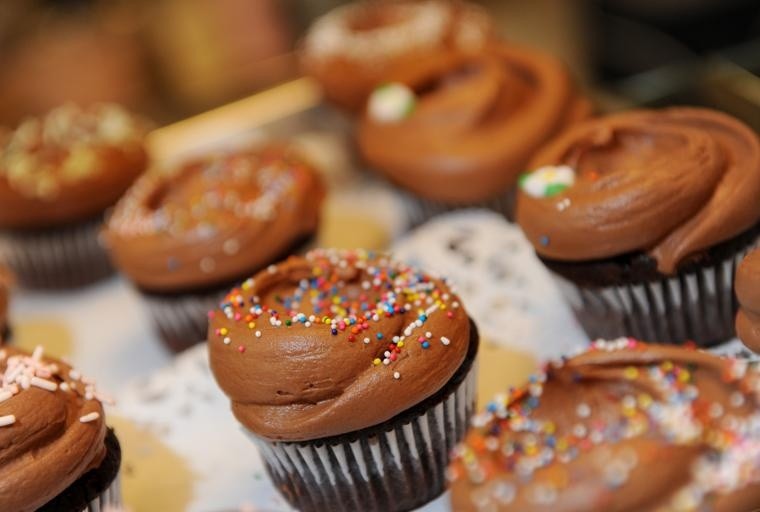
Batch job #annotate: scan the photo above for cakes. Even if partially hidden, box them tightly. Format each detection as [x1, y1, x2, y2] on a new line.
[1, 0, 760, 511]
